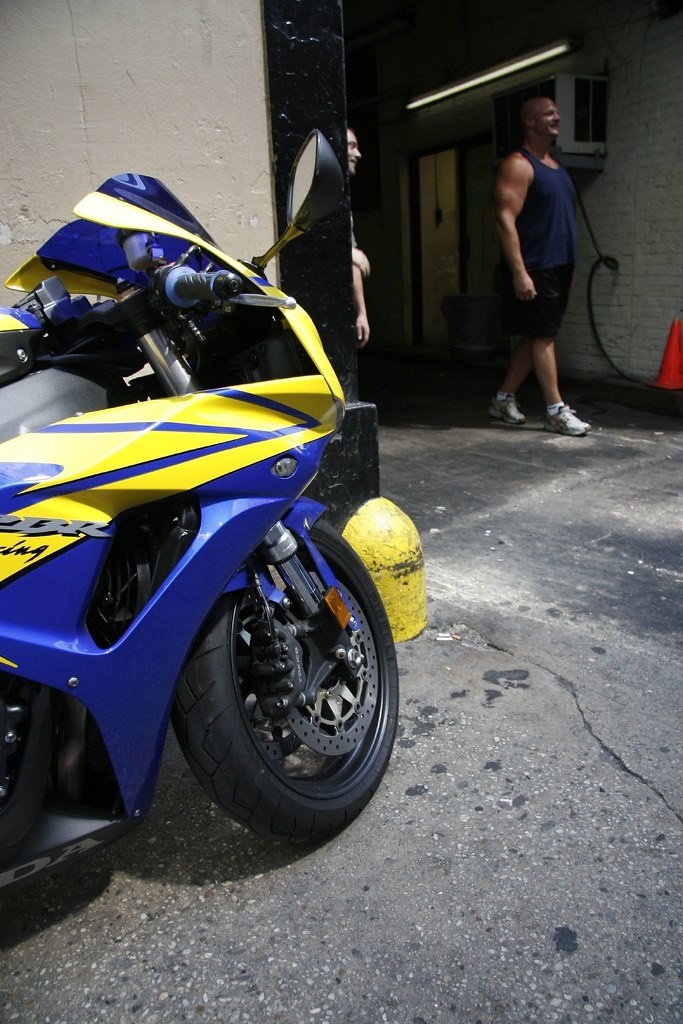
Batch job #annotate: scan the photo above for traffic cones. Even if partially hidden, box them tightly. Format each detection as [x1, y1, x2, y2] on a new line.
[646, 318, 683, 391]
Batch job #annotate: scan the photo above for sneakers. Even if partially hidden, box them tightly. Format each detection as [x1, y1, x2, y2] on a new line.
[488, 395, 526, 424]
[545, 404, 592, 435]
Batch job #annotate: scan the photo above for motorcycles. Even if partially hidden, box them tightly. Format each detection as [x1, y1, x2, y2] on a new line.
[0, 130, 400, 898]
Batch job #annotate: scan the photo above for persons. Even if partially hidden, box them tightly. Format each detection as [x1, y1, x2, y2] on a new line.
[490, 97, 593, 435]
[346, 125, 372, 348]
